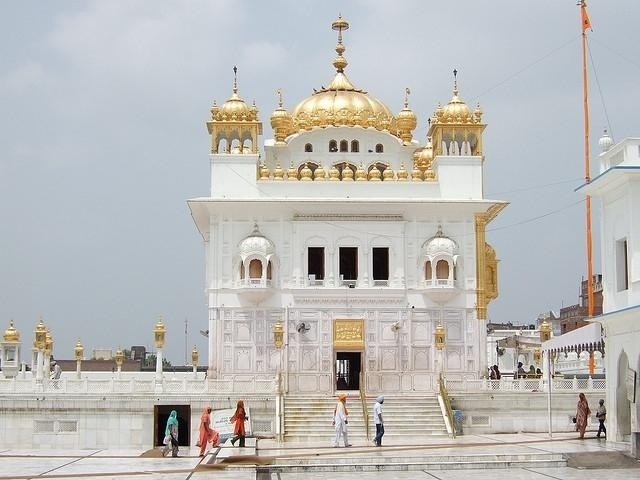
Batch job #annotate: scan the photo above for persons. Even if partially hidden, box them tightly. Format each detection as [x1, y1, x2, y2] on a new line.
[596, 398, 606, 438]
[333, 393, 352, 447]
[372, 394, 385, 448]
[50, 361, 62, 389]
[488, 362, 541, 389]
[230, 400, 248, 448]
[576, 393, 589, 439]
[195, 406, 220, 458]
[160, 411, 180, 458]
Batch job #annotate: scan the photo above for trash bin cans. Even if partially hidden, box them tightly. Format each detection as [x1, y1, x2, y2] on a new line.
[451, 409, 464, 436]
